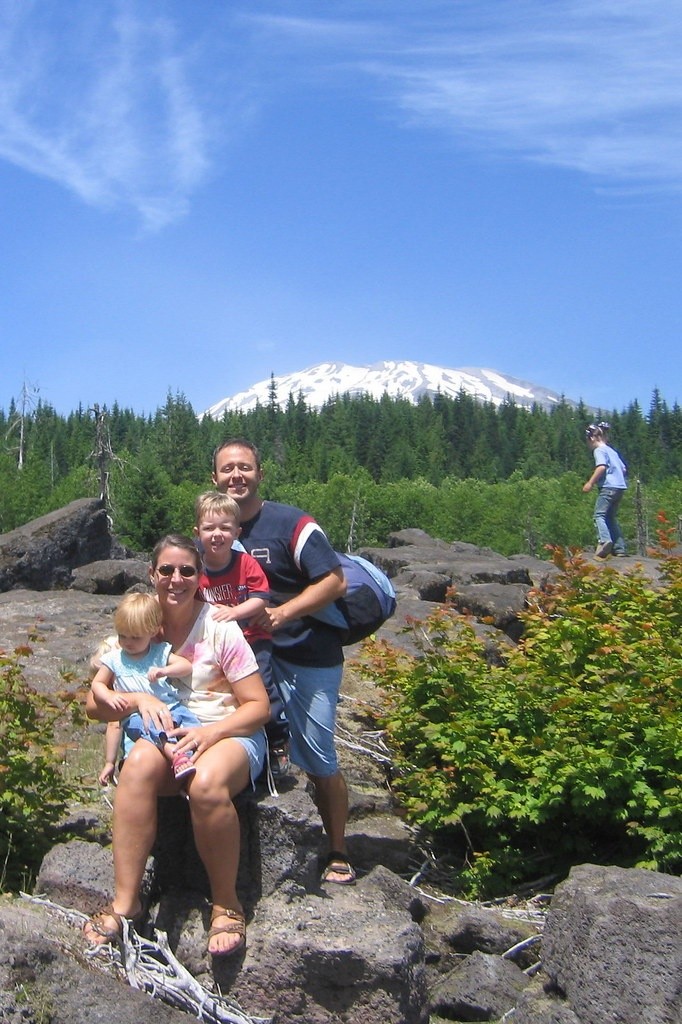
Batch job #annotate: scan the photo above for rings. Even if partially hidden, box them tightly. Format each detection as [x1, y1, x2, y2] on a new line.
[194, 741, 197, 747]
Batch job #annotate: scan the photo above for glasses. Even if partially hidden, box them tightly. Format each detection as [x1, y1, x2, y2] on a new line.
[153, 564, 198, 578]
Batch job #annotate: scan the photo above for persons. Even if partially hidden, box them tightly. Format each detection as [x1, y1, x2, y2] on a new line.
[87, 533, 272, 956]
[194, 492, 269, 694]
[194, 441, 350, 885]
[581, 422, 628, 557]
[92, 592, 203, 777]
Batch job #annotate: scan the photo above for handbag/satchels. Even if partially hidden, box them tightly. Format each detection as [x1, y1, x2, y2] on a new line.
[334, 551, 396, 646]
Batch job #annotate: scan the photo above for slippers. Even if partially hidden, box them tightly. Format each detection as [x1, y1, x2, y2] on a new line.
[83, 897, 147, 944]
[207, 908, 246, 957]
[321, 851, 356, 885]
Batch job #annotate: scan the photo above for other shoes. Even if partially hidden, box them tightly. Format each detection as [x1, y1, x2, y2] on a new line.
[613, 543, 626, 556]
[595, 542, 613, 558]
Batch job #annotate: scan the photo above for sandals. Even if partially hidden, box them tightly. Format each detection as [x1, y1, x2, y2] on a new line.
[270, 745, 288, 778]
[172, 754, 196, 780]
